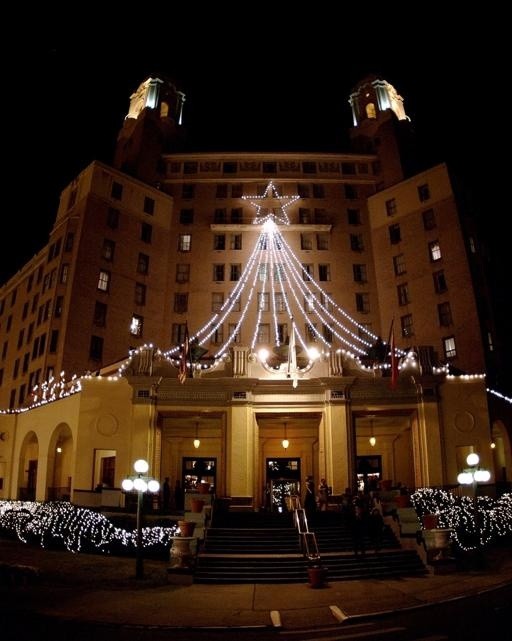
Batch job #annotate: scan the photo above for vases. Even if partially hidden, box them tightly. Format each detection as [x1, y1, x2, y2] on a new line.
[394, 495, 410, 508]
[306, 568, 331, 590]
[421, 513, 440, 530]
[197, 482, 211, 496]
[176, 520, 198, 537]
[190, 498, 205, 515]
[379, 478, 394, 492]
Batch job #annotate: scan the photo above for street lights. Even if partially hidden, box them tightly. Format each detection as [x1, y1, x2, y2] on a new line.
[457, 452, 490, 573]
[122, 459, 162, 578]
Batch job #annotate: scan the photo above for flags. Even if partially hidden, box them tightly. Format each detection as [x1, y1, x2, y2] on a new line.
[387, 326, 400, 389]
[178, 326, 190, 384]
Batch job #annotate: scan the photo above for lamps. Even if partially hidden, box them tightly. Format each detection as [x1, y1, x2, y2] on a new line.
[193, 422, 201, 449]
[366, 419, 379, 449]
[56, 440, 65, 454]
[489, 433, 497, 451]
[280, 422, 292, 453]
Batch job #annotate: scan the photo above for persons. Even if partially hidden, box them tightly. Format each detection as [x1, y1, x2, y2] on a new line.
[303, 475, 316, 511]
[318, 478, 334, 511]
